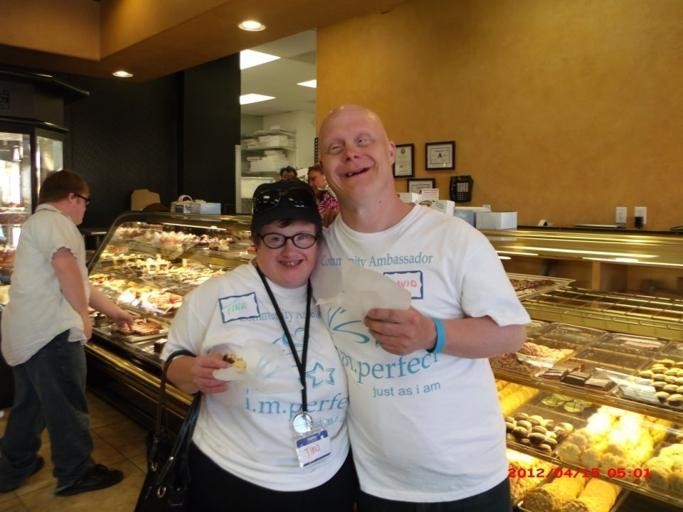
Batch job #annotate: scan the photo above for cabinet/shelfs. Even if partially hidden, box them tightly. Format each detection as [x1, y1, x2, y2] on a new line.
[241, 130, 297, 177]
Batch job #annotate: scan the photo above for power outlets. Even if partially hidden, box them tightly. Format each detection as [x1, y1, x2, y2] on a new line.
[635, 207, 647, 224]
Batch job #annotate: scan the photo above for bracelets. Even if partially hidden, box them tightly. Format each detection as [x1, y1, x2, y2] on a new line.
[427, 317, 445, 354]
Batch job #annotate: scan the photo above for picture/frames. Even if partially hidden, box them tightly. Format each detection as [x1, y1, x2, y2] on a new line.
[425, 141, 455, 170]
[393, 143, 414, 177]
[407, 178, 435, 193]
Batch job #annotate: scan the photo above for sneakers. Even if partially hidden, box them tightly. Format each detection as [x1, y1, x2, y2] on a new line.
[56, 464, 123, 496]
[0, 456, 45, 492]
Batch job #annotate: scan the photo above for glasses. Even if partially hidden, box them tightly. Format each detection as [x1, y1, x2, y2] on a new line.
[255, 189, 312, 211]
[258, 231, 320, 249]
[74, 193, 92, 206]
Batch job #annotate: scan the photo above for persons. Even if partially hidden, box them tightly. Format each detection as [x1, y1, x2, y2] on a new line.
[1, 172, 134, 498]
[308, 165, 340, 225]
[308, 105, 531, 512]
[280, 167, 297, 181]
[161, 177, 360, 512]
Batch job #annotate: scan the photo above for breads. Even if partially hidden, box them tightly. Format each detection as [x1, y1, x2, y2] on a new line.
[505, 359, 683, 512]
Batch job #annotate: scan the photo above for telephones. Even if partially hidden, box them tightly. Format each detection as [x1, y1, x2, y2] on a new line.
[449, 175, 473, 202]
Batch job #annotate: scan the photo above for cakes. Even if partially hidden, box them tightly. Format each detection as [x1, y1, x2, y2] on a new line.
[222, 354, 247, 372]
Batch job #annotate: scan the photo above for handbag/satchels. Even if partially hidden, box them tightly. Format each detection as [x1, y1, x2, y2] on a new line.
[134, 430, 189, 512]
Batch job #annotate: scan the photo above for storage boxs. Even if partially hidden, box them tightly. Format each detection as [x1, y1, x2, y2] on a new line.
[476, 212, 518, 229]
[454, 207, 489, 227]
[192, 203, 221, 214]
[396, 193, 421, 202]
[170, 200, 206, 213]
[416, 200, 455, 216]
